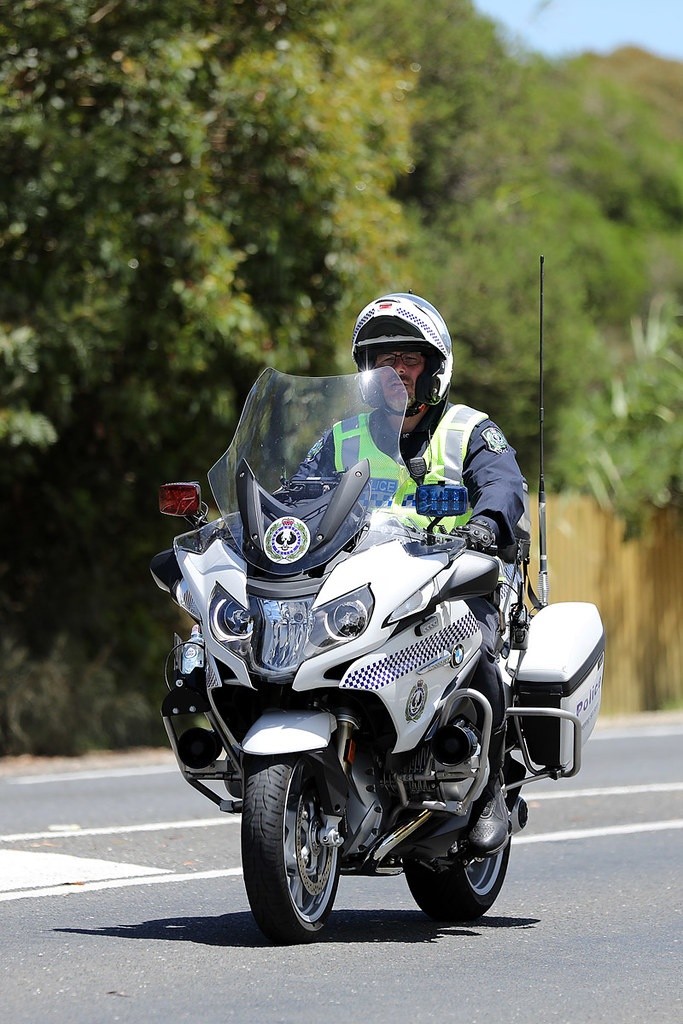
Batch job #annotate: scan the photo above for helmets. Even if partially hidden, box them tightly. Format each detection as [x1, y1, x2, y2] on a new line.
[350, 290, 453, 417]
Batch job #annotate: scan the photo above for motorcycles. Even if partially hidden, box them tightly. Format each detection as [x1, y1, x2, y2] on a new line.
[146, 252, 608, 947]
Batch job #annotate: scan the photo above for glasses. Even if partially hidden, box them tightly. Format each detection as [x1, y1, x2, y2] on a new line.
[372, 352, 423, 368]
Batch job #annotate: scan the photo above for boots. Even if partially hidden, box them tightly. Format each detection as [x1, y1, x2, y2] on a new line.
[467, 712, 514, 857]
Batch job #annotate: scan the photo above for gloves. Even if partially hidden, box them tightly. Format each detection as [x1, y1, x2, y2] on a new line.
[444, 516, 498, 553]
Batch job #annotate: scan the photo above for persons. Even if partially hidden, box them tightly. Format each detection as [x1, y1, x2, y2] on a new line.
[282, 289, 528, 858]
